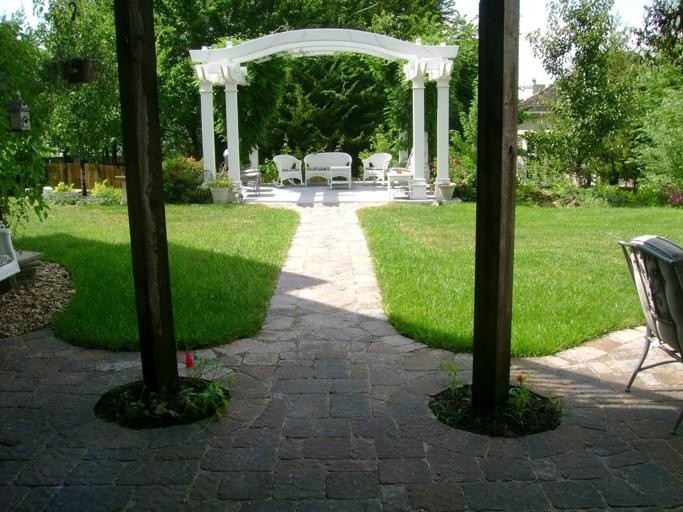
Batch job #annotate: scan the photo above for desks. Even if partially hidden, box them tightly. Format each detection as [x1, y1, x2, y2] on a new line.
[114, 176, 127, 206]
[328, 165, 352, 190]
[386, 170, 413, 201]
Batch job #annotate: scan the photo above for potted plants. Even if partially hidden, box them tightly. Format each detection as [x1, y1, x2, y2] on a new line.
[208, 177, 232, 204]
[439, 180, 458, 200]
[39, 22, 112, 84]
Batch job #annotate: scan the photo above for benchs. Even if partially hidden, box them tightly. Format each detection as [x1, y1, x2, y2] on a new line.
[303, 151, 353, 187]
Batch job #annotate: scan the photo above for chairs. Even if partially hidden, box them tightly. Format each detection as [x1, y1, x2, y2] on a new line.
[270, 154, 305, 188]
[360, 152, 393, 188]
[0, 226, 21, 282]
[219, 149, 261, 197]
[617, 233, 683, 435]
